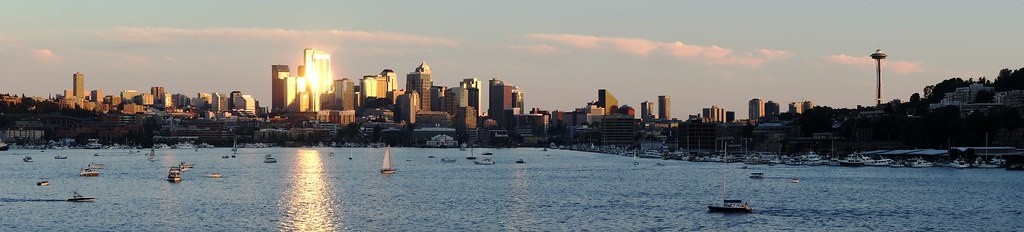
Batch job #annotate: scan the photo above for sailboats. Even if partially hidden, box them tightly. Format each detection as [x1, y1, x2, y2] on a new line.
[707, 139, 755, 215]
[379, 144, 398, 175]
[348, 148, 353, 160]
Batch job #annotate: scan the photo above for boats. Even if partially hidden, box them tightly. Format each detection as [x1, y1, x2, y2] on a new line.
[549, 141, 1024, 171]
[750, 172, 765, 179]
[791, 179, 800, 183]
[428, 146, 526, 166]
[0, 141, 277, 203]
[327, 152, 335, 157]
